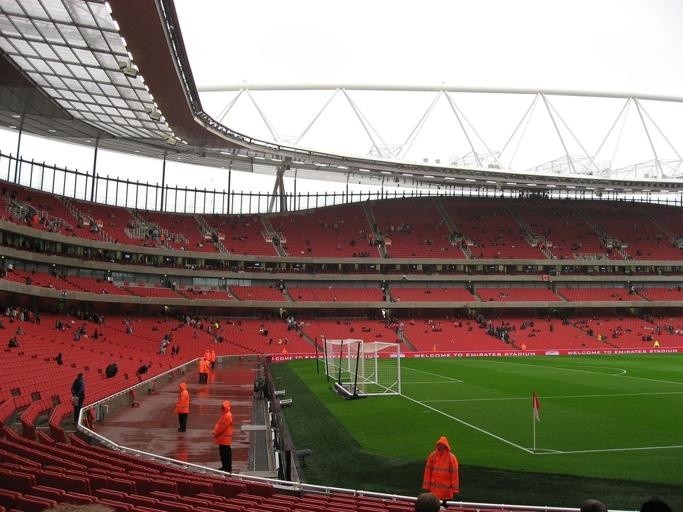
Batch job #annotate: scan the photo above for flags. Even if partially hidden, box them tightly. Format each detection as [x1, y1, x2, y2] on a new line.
[532, 389, 540, 423]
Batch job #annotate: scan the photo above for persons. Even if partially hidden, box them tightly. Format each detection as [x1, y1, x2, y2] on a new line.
[197, 358, 207, 384]
[422, 436, 460, 501]
[414, 492, 449, 512]
[212, 399, 232, 477]
[640, 497, 674, 511]
[578, 499, 609, 511]
[203, 358, 209, 384]
[173, 382, 190, 432]
[69, 372, 87, 426]
[1, 186, 682, 379]
